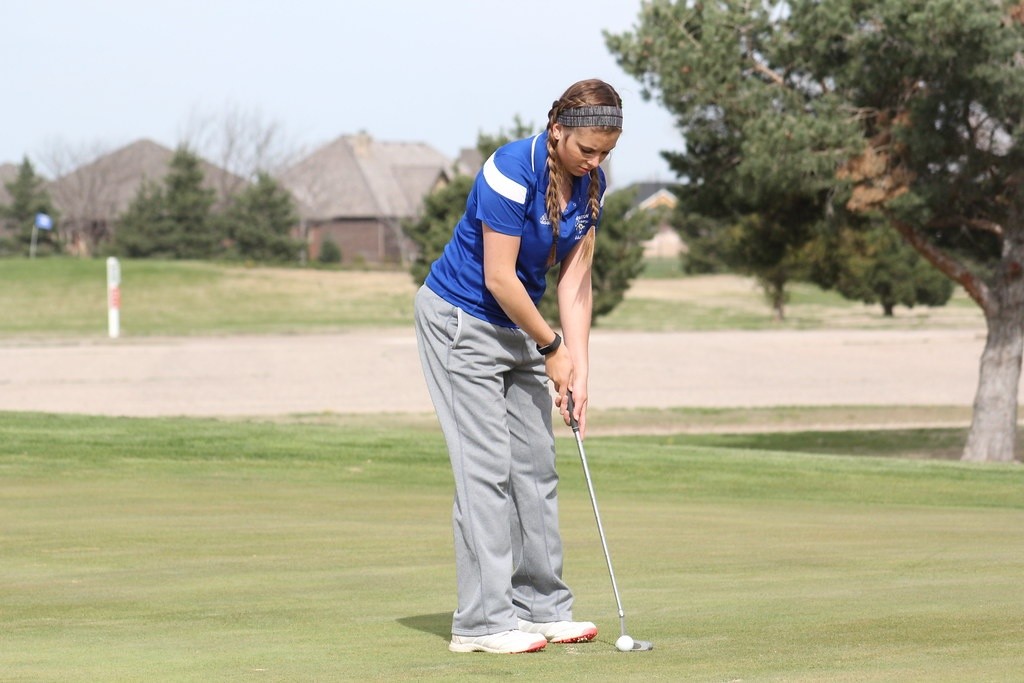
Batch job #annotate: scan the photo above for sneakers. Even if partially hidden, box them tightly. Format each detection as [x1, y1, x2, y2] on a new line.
[448, 629, 548, 654]
[517, 618, 598, 644]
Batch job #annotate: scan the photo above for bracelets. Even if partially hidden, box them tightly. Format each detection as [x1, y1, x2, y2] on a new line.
[536, 332, 561, 355]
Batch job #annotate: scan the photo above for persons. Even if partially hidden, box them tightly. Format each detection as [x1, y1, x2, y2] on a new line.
[413, 79, 623, 653]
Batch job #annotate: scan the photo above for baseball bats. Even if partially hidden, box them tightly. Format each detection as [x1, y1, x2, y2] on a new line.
[560, 377, 655, 654]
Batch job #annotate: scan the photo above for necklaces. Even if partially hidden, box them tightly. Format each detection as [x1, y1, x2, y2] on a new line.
[559, 185, 566, 203]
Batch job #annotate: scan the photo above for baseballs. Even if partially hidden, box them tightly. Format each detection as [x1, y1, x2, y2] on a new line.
[615, 634, 634, 653]
